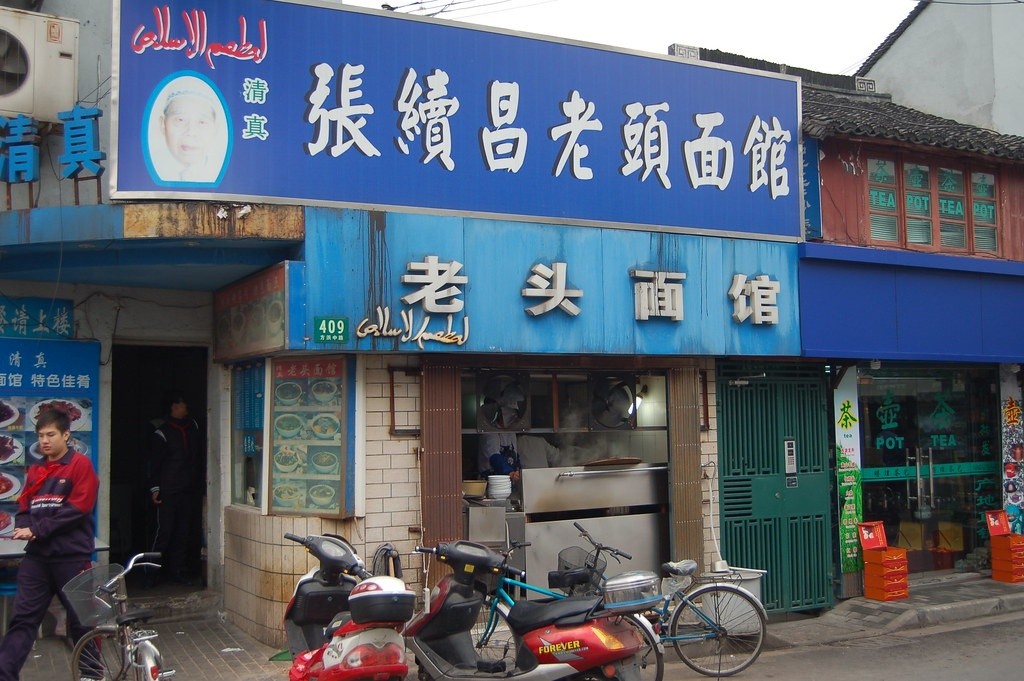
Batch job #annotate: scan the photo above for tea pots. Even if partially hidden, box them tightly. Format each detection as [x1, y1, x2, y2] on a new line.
[1004, 445, 1023, 505]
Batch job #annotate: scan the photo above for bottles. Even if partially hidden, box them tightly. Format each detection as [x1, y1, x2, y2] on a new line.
[866, 493, 873, 513]
[882, 494, 889, 512]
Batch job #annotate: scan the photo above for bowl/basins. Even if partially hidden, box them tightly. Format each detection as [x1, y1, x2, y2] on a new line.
[463, 480, 487, 496]
[274, 450, 299, 472]
[312, 381, 337, 401]
[275, 414, 303, 438]
[488, 475, 512, 499]
[275, 382, 302, 405]
[312, 413, 340, 438]
[311, 452, 337, 473]
[309, 485, 335, 505]
[273, 485, 301, 506]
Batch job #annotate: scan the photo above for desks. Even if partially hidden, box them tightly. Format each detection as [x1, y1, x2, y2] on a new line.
[0, 537, 110, 557]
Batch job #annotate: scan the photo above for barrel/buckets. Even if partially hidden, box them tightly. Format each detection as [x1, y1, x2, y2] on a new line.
[700, 571, 763, 635]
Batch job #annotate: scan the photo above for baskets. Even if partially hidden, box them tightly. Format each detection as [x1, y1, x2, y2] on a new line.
[62, 563, 127, 627]
[557, 546, 607, 598]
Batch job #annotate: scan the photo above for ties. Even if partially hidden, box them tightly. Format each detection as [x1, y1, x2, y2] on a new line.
[166, 419, 192, 450]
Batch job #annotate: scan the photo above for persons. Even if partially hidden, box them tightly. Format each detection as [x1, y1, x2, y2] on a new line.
[477, 376, 560, 486]
[142, 393, 199, 590]
[0, 409, 104, 681]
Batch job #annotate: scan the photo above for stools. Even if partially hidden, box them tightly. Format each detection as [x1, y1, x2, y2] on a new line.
[0, 568, 42, 640]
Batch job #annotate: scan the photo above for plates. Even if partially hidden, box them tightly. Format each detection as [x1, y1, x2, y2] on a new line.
[29, 399, 87, 431]
[0, 435, 23, 464]
[0, 473, 21, 499]
[0, 402, 20, 429]
[30, 437, 88, 459]
[0, 517, 15, 534]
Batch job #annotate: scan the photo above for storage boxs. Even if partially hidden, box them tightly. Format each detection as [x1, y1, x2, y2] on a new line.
[857, 521, 908, 601]
[985, 510, 1024, 583]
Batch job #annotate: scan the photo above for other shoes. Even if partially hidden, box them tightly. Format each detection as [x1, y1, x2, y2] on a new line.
[164, 567, 185, 585]
[131, 574, 164, 590]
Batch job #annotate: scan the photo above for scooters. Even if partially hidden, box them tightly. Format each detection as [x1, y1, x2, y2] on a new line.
[281, 531, 418, 680]
[402, 539, 665, 681]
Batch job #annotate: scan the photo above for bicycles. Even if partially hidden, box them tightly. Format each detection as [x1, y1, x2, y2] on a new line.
[470, 538, 666, 681]
[556, 521, 768, 681]
[60, 551, 177, 681]
